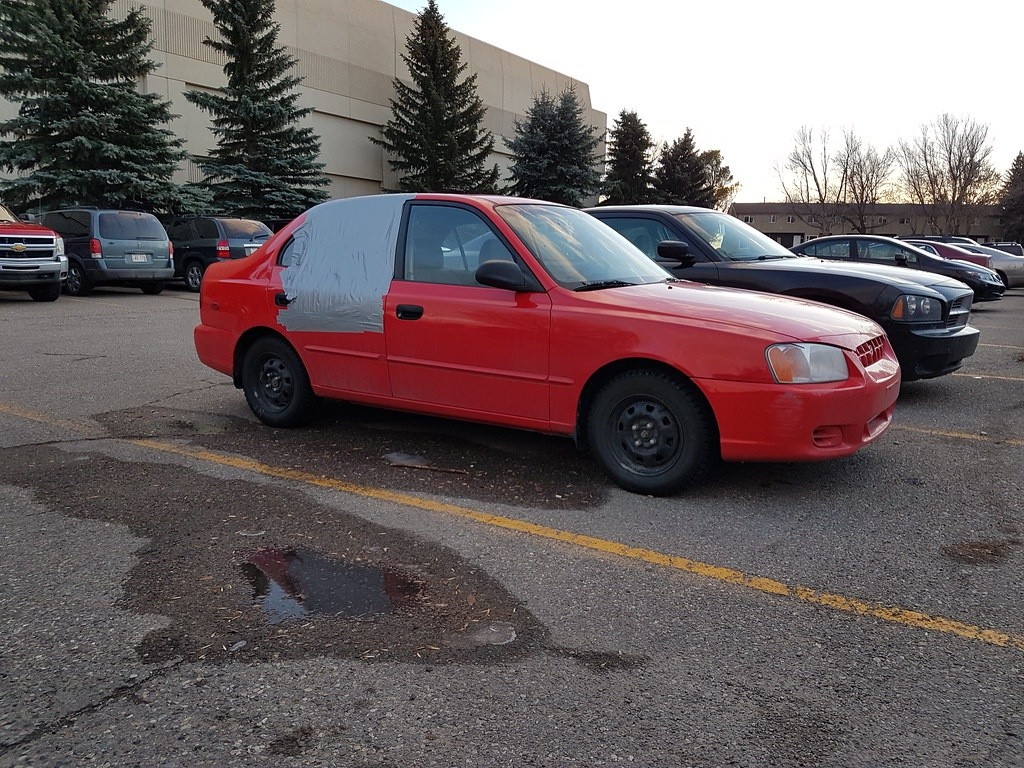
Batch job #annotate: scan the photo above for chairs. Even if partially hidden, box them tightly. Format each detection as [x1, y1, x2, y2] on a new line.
[633, 235, 650, 254]
[414, 238, 460, 285]
[469, 238, 513, 285]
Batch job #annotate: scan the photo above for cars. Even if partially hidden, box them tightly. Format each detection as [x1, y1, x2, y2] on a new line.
[195, 193, 900, 495]
[905, 243, 1024, 289]
[843, 240, 993, 270]
[787, 235, 1006, 304]
[440, 204, 980, 382]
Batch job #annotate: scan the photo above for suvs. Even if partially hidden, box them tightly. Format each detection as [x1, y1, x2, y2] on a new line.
[167, 214, 275, 292]
[893, 236, 979, 246]
[980, 242, 1024, 256]
[25, 206, 176, 296]
[0, 201, 69, 301]
